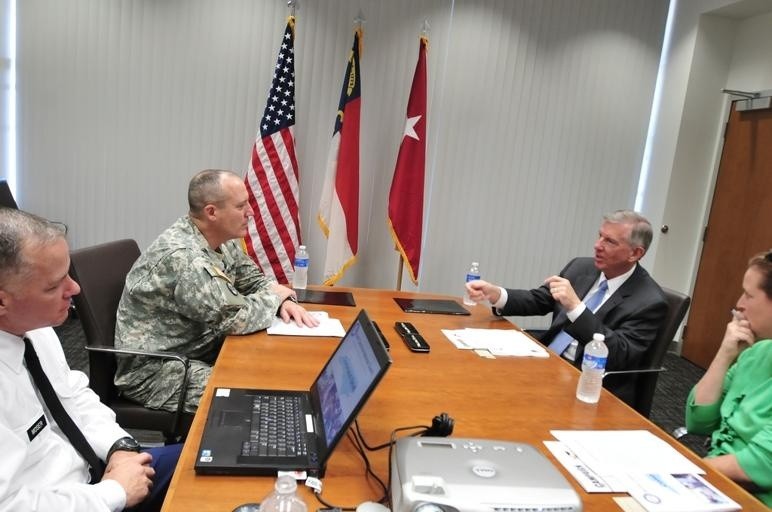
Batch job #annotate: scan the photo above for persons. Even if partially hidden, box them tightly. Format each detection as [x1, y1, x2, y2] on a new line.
[113, 169, 320, 414]
[0, 207, 184, 512]
[465, 211, 671, 406]
[684, 250, 772, 508]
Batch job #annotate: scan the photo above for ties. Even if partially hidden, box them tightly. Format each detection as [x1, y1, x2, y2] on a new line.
[24, 338, 103, 481]
[547, 280, 608, 355]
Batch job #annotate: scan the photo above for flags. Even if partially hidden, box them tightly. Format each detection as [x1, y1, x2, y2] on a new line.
[381, 21, 427, 293]
[236, 0, 302, 287]
[316, 16, 363, 288]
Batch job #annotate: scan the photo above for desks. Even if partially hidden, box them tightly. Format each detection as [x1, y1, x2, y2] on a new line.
[160, 283, 772, 512]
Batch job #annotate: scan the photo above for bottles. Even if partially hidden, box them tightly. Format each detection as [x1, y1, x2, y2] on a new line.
[259, 475, 308, 511]
[291, 245, 309, 290]
[576, 333, 608, 403]
[462, 262, 480, 306]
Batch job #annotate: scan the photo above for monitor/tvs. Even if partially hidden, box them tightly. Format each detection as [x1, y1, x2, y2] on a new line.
[193, 309, 394, 479]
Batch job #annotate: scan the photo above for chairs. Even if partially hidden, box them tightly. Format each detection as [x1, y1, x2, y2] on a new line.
[671, 425, 717, 449]
[69, 237, 195, 447]
[522, 284, 691, 421]
[0, 179, 76, 316]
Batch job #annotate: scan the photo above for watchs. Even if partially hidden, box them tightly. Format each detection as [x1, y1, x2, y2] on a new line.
[287, 294, 296, 302]
[106, 437, 141, 461]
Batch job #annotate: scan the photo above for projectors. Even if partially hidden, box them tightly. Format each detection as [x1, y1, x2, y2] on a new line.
[391, 436, 583, 511]
